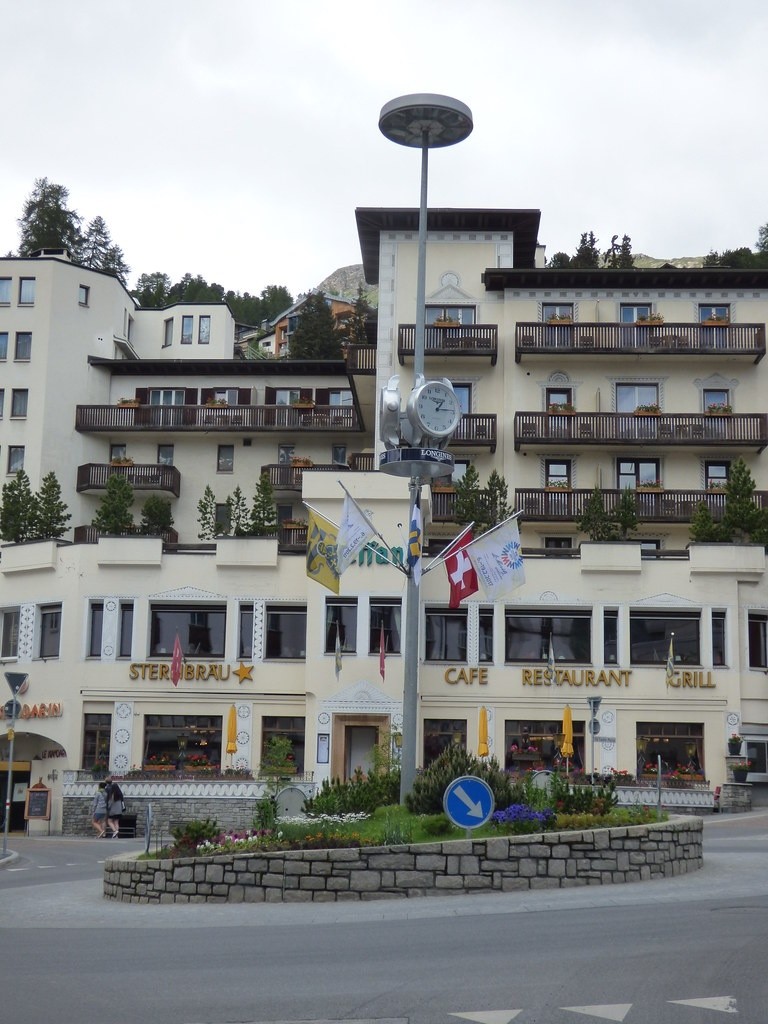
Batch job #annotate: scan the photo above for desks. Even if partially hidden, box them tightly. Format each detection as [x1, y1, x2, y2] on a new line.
[679, 500, 695, 516]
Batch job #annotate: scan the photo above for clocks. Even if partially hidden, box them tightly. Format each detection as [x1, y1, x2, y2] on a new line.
[407, 372, 462, 438]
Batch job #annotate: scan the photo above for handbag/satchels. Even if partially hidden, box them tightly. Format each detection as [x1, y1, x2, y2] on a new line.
[121, 801, 126, 812]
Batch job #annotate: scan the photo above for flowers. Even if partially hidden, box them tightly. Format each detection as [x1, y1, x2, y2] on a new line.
[434, 311, 724, 322]
[511, 734, 752, 783]
[282, 517, 307, 526]
[117, 397, 317, 406]
[144, 756, 298, 767]
[291, 456, 312, 464]
[546, 479, 727, 489]
[548, 402, 732, 411]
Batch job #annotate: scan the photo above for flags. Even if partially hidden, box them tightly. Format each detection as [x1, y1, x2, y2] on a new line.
[465, 517, 526, 602]
[333, 631, 343, 682]
[170, 638, 182, 687]
[443, 530, 479, 609]
[404, 493, 422, 587]
[305, 508, 340, 597]
[546, 643, 557, 687]
[378, 627, 386, 682]
[335, 492, 375, 576]
[665, 639, 675, 690]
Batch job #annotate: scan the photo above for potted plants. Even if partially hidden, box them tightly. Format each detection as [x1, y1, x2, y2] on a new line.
[195, 471, 280, 567]
[91, 473, 175, 566]
[111, 456, 133, 467]
[687, 454, 768, 573]
[0, 469, 73, 573]
[574, 483, 643, 571]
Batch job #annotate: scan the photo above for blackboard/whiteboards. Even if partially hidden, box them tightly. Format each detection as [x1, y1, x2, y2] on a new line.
[24, 789, 51, 820]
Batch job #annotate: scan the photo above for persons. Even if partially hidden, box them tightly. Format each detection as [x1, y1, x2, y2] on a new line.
[91, 777, 126, 839]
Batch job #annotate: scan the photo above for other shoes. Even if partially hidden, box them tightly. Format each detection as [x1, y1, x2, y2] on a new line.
[97, 830, 105, 838]
[102, 834, 106, 838]
[112, 830, 119, 838]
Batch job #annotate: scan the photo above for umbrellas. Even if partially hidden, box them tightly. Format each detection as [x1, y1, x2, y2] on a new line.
[227, 704, 238, 768]
[561, 704, 574, 773]
[477, 706, 489, 762]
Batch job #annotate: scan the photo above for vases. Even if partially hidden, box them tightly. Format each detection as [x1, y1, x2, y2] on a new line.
[144, 764, 299, 774]
[117, 403, 315, 408]
[282, 523, 308, 529]
[431, 488, 728, 494]
[547, 410, 732, 417]
[434, 319, 732, 326]
[291, 463, 313, 467]
[732, 769, 749, 783]
[728, 742, 742, 756]
